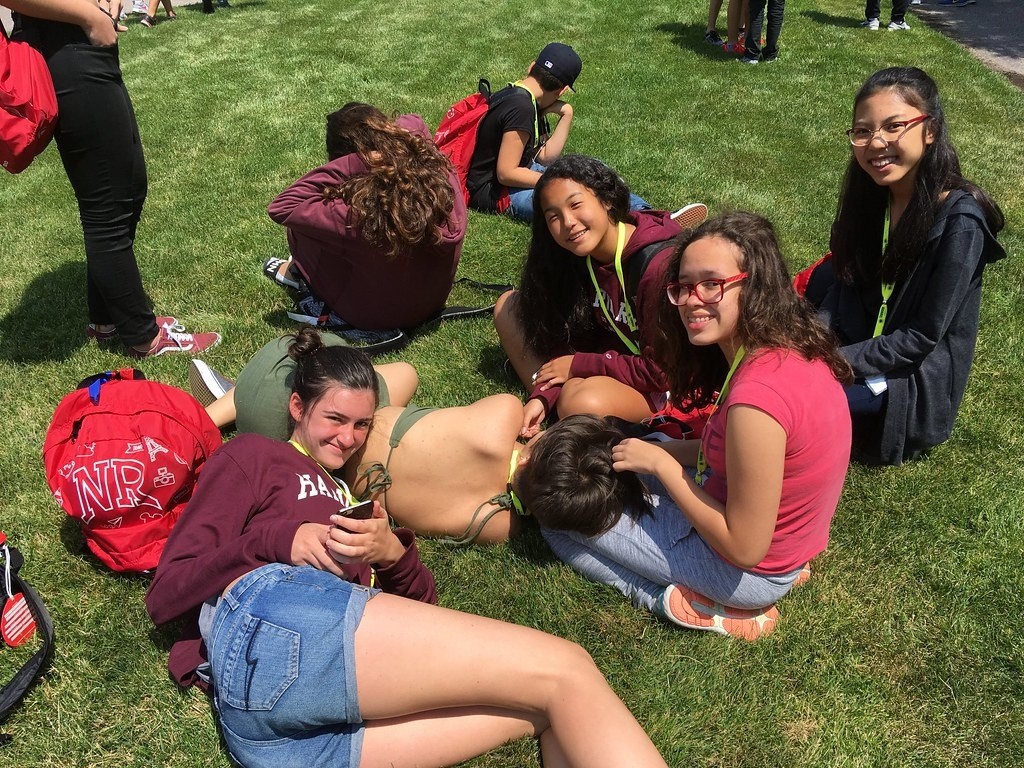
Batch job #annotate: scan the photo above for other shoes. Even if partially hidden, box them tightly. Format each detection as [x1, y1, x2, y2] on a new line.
[188, 359, 237, 407]
[860, 18, 879, 30]
[722, 41, 746, 55]
[765, 57, 777, 64]
[791, 560, 811, 584]
[740, 36, 765, 47]
[736, 56, 758, 65]
[887, 20, 910, 31]
[739, 28, 745, 34]
[661, 582, 779, 642]
[703, 30, 723, 46]
[911, 0, 921, 4]
[263, 256, 300, 291]
[670, 203, 709, 231]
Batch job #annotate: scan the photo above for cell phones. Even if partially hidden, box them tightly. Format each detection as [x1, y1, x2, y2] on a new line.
[333, 500, 375, 534]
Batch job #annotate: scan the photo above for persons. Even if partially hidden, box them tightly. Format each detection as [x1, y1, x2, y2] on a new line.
[861, 0, 913, 31]
[805, 66, 1007, 463]
[494, 156, 686, 436]
[263, 104, 469, 348]
[132, 0, 176, 28]
[542, 210, 851, 640]
[190, 332, 653, 548]
[146, 329, 668, 768]
[705, 0, 786, 65]
[465, 43, 708, 231]
[202, 0, 231, 14]
[0, 0, 221, 360]
[98, 0, 128, 31]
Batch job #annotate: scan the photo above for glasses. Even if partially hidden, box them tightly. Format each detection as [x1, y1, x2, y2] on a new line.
[665, 271, 751, 306]
[846, 114, 930, 147]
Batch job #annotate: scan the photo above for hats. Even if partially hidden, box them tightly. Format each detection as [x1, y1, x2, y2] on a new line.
[536, 42, 582, 93]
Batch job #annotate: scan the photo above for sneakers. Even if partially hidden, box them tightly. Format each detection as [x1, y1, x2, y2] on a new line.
[87, 314, 178, 344]
[127, 325, 222, 360]
[120, 0, 231, 28]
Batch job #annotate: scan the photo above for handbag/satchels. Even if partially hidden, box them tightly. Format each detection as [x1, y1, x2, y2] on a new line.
[0, 18, 60, 175]
[287, 274, 514, 357]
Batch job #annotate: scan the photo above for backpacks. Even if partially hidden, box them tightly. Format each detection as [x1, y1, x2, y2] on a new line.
[433, 79, 531, 209]
[43, 369, 224, 577]
